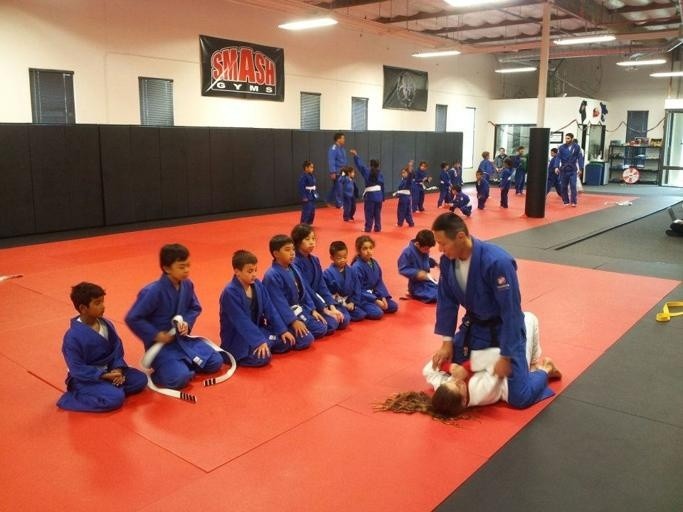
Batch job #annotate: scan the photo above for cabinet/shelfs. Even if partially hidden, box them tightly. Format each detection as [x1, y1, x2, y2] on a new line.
[609, 145, 661, 184]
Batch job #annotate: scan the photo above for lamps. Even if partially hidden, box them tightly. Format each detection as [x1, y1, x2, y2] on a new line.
[412, 50, 462, 58]
[495, 68, 538, 73]
[555, 34, 617, 45]
[616, 58, 668, 67]
[277, 12, 339, 31]
[648, 71, 683, 77]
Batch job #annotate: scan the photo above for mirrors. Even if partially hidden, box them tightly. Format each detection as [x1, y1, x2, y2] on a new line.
[492, 123, 537, 163]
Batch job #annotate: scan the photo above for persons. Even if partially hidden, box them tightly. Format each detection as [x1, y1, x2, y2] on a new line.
[219, 225, 398, 368]
[123, 243, 224, 390]
[56, 281, 148, 413]
[476, 133, 585, 209]
[396, 168, 414, 227]
[407, 159, 472, 217]
[337, 167, 358, 222]
[397, 229, 440, 303]
[297, 160, 317, 224]
[422, 312, 542, 417]
[324, 133, 347, 210]
[350, 149, 385, 232]
[431, 213, 563, 410]
